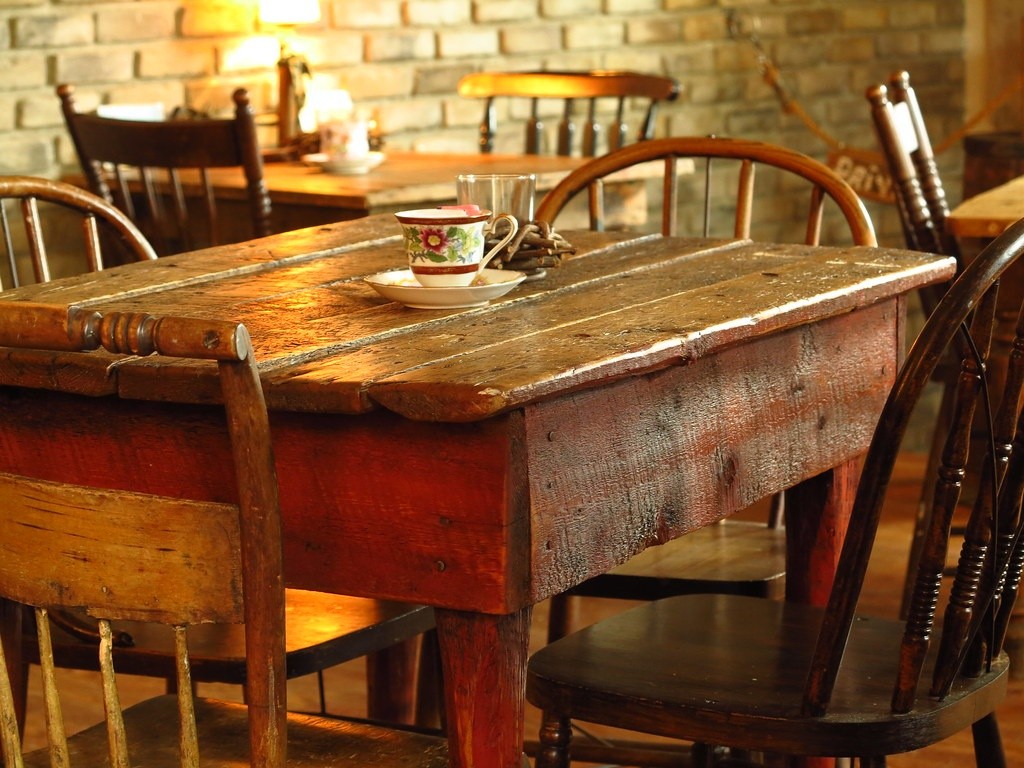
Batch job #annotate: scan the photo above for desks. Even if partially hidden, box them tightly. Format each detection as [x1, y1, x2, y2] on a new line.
[0, 207, 1024, 768]
[54, 144, 696, 266]
[942, 177, 1024, 618]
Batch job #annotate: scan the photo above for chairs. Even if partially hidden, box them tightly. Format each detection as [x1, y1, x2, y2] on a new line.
[0, 70, 1024, 768]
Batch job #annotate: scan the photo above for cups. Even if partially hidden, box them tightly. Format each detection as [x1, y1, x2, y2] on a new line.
[455, 174, 537, 227]
[393, 204, 519, 287]
[318, 122, 369, 158]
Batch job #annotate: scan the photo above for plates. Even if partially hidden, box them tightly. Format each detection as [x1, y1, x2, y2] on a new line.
[301, 152, 385, 175]
[362, 269, 527, 309]
